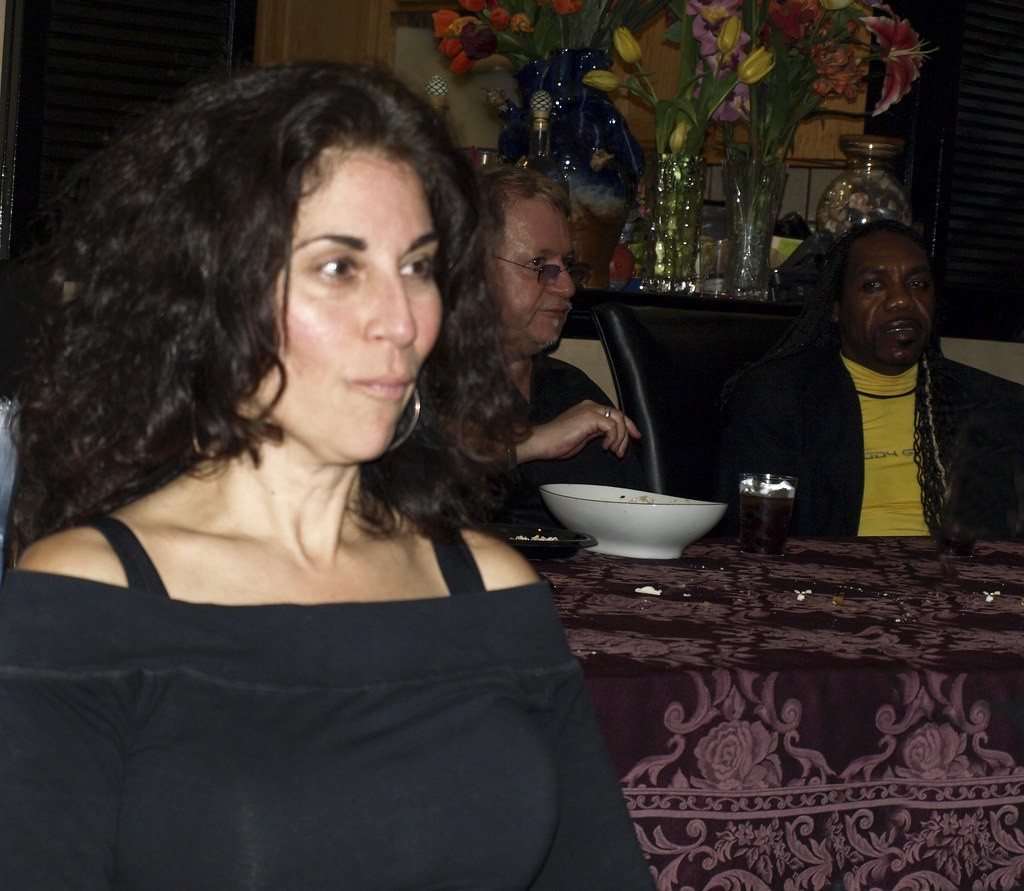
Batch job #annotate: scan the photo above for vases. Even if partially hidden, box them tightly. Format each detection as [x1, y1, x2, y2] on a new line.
[640, 153, 706, 295]
[815, 134, 913, 252]
[495, 48, 644, 290]
[720, 158, 789, 302]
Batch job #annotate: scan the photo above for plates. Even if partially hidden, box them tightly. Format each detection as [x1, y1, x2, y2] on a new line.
[477, 523, 598, 560]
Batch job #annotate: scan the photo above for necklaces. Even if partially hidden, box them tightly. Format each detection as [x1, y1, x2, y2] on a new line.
[857, 386, 916, 399]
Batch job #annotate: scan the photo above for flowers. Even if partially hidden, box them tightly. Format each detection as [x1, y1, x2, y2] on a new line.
[583, 1, 778, 156]
[432, 1, 670, 75]
[682, 1, 940, 156]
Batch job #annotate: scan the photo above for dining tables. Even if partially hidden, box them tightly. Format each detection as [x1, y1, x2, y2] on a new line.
[522, 531, 1023, 891]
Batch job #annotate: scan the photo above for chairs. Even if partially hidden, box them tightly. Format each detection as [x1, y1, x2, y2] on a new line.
[589, 302, 834, 532]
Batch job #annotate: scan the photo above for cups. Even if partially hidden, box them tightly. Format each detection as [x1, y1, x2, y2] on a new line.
[738, 472, 799, 562]
[700, 214, 729, 297]
[936, 479, 985, 561]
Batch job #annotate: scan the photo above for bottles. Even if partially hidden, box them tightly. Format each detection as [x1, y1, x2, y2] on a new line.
[513, 89, 570, 224]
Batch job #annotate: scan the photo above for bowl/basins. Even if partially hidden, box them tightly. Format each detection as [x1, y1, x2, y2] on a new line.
[540, 483, 728, 559]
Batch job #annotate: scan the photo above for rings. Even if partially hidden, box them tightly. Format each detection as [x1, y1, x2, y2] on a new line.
[606, 407, 611, 417]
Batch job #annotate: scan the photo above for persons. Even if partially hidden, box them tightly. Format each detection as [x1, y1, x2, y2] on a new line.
[721, 219, 1024, 539]
[0, 60, 653, 891]
[454, 162, 648, 522]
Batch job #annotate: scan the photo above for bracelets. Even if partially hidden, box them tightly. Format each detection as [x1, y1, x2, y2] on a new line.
[506, 445, 517, 472]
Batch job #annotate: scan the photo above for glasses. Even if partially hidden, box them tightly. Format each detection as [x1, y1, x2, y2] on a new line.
[490, 253, 593, 285]
